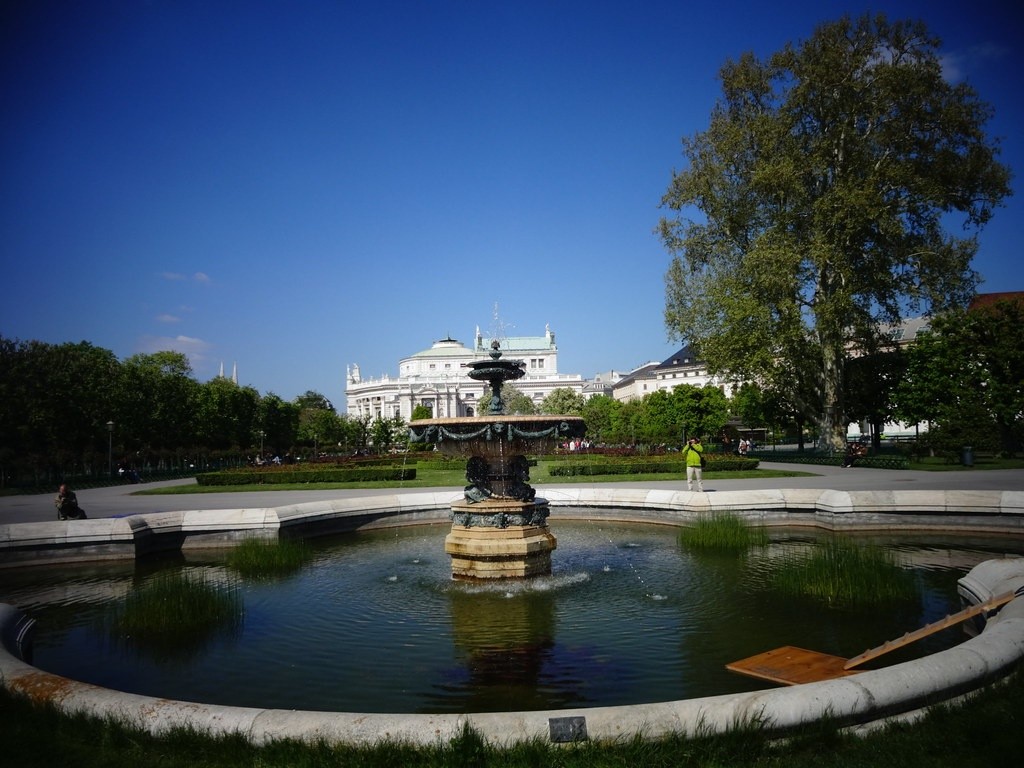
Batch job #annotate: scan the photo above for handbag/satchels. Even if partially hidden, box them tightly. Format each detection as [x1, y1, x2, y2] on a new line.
[700, 456, 707, 468]
[741, 443, 746, 451]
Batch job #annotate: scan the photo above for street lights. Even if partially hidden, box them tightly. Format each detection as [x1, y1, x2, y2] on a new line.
[259, 430, 265, 462]
[106, 420, 114, 478]
[344, 436, 349, 452]
[313, 434, 318, 460]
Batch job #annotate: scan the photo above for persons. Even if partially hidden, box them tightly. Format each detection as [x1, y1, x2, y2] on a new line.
[55, 486, 88, 521]
[722, 434, 867, 469]
[558, 436, 595, 451]
[682, 437, 704, 492]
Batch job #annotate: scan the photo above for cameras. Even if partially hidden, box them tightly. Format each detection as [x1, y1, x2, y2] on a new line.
[689, 442, 693, 444]
